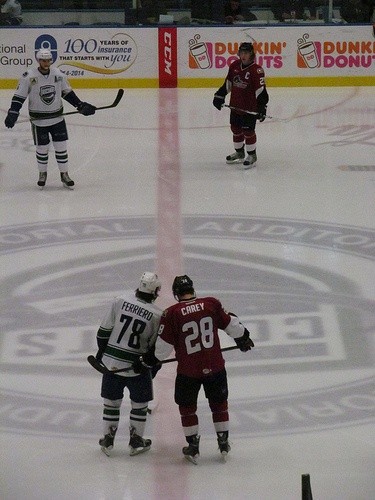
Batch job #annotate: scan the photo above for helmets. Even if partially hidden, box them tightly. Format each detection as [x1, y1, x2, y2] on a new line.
[36, 49, 52, 60]
[172, 274, 195, 302]
[238, 43, 254, 56]
[138, 271, 162, 299]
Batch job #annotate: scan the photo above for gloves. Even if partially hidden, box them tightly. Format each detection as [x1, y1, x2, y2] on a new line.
[213, 96, 225, 111]
[235, 336, 255, 352]
[77, 102, 97, 116]
[4, 111, 20, 128]
[149, 356, 162, 378]
[254, 106, 268, 122]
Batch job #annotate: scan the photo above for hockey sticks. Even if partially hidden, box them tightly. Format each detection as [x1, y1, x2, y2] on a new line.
[13, 89, 124, 123]
[87, 344, 239, 375]
[223, 105, 301, 124]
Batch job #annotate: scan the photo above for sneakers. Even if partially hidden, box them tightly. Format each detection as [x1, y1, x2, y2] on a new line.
[226, 151, 245, 164]
[129, 426, 152, 456]
[60, 171, 75, 190]
[217, 431, 231, 461]
[37, 171, 47, 190]
[243, 153, 257, 168]
[98, 427, 118, 457]
[182, 434, 201, 465]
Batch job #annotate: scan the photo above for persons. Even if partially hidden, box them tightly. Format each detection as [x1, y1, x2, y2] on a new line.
[97, 272, 164, 457]
[0, 0, 22, 25]
[342, 0, 374, 23]
[213, 41, 269, 169]
[214, 0, 256, 22]
[132, 274, 255, 465]
[4, 49, 96, 190]
[271, 0, 316, 20]
